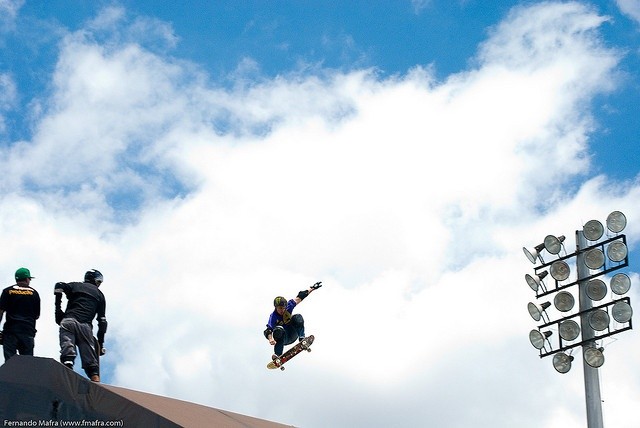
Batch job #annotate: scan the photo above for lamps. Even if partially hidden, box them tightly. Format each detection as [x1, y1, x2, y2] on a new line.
[583, 347, 606, 367]
[582, 220, 604, 240]
[529, 329, 552, 349]
[553, 352, 575, 373]
[588, 310, 610, 331]
[584, 249, 606, 270]
[525, 270, 549, 292]
[522, 243, 545, 264]
[607, 239, 628, 262]
[607, 211, 626, 233]
[612, 302, 633, 323]
[610, 274, 631, 296]
[585, 278, 607, 301]
[554, 291, 575, 312]
[544, 235, 566, 254]
[549, 261, 570, 281]
[559, 320, 580, 341]
[527, 301, 551, 321]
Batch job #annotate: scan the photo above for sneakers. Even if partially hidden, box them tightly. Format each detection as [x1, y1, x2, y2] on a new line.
[91, 375, 99, 381]
[272, 354, 279, 361]
[299, 338, 305, 341]
[61, 358, 74, 366]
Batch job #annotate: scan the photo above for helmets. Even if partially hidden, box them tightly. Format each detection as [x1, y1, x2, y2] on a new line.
[274, 296, 287, 308]
[84, 268, 103, 285]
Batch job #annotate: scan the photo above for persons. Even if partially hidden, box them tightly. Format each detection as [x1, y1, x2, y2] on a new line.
[263, 281, 322, 361]
[0, 267, 41, 363]
[53, 268, 107, 382]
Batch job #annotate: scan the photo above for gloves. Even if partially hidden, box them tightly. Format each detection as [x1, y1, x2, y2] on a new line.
[309, 282, 322, 290]
[99, 342, 105, 356]
[55, 311, 66, 325]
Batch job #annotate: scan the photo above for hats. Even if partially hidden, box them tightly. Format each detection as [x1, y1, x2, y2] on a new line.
[15, 267, 35, 280]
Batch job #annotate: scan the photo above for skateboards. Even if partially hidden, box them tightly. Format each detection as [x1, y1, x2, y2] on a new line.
[267, 335, 315, 370]
